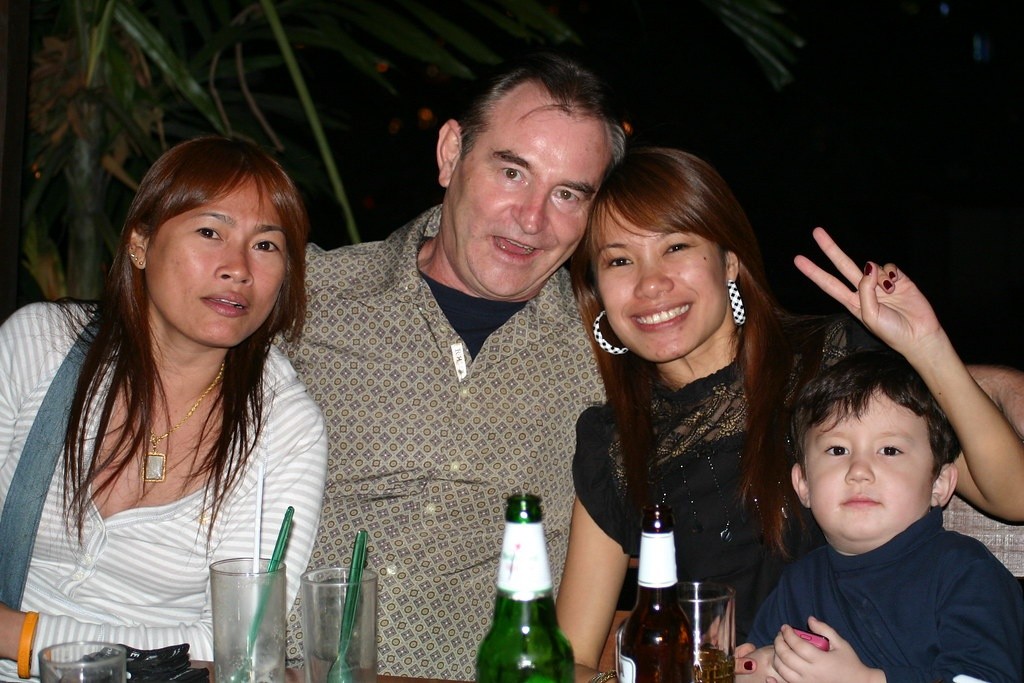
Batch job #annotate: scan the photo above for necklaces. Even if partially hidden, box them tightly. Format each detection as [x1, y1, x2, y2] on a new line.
[135, 361, 223, 482]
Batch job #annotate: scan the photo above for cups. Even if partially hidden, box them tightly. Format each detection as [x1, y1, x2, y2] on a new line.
[209, 557, 286, 683]
[675, 581, 737, 683]
[300, 566, 377, 683]
[38, 642, 127, 683]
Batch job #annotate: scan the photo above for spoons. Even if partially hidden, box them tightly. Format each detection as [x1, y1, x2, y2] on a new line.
[227, 506, 295, 683]
[325, 527, 369, 683]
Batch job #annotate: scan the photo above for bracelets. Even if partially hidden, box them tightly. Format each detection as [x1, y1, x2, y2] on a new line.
[589, 669, 617, 683]
[17, 612, 38, 679]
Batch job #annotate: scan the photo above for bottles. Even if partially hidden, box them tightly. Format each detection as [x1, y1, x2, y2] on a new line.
[474, 493, 576, 683]
[619, 501, 694, 683]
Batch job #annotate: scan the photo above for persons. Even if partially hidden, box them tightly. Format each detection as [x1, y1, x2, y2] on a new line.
[739, 348, 1024, 683]
[552, 151, 1024, 683]
[269, 62, 632, 683]
[0, 134, 330, 683]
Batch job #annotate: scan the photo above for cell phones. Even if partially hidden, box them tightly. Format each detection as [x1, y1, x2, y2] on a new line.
[792, 628, 830, 652]
